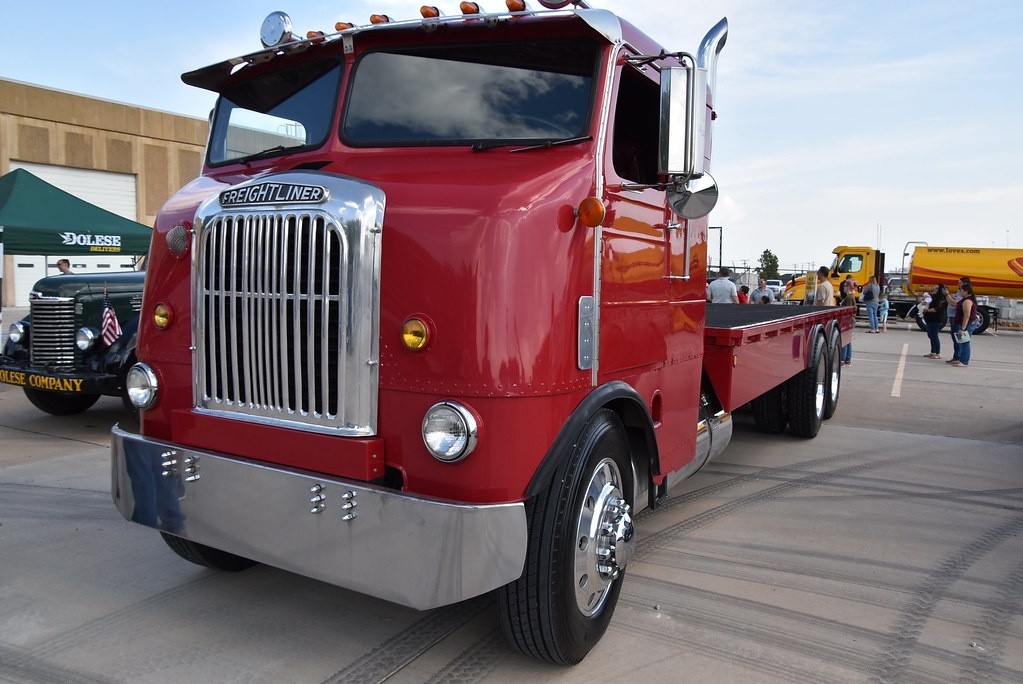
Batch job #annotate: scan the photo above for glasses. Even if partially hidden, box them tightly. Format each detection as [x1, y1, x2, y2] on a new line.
[758, 281, 766, 284]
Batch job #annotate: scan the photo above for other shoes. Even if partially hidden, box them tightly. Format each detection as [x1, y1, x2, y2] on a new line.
[952, 362, 967, 366]
[876, 330, 880, 333]
[865, 330, 875, 333]
[923, 353, 937, 357]
[841, 361, 845, 367]
[946, 358, 960, 364]
[930, 354, 942, 359]
[882, 329, 887, 333]
[845, 361, 851, 366]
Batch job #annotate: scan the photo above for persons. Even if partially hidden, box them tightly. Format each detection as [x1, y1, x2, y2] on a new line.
[839, 279, 857, 367]
[737, 286, 749, 304]
[706, 267, 739, 304]
[859, 276, 880, 333]
[878, 293, 889, 332]
[749, 277, 774, 304]
[941, 277, 977, 367]
[57, 258, 72, 273]
[917, 283, 948, 359]
[839, 274, 853, 297]
[816, 267, 833, 305]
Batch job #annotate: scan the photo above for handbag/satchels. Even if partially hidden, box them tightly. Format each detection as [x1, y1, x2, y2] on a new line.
[858, 292, 865, 302]
[864, 283, 873, 300]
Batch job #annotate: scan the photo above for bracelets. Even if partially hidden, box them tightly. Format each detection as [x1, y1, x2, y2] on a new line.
[960, 328, 966, 331]
[945, 294, 948, 297]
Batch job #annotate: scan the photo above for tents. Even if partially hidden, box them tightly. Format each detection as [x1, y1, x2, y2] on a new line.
[0, 168, 154, 326]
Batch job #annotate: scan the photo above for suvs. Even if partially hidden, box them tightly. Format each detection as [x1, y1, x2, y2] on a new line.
[765, 280, 784, 301]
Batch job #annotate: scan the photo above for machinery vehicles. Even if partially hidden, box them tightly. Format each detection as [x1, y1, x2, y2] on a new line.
[0, 251, 150, 415]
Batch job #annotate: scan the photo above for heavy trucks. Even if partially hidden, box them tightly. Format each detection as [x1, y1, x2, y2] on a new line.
[106, 1, 860, 667]
[781, 241, 1023, 334]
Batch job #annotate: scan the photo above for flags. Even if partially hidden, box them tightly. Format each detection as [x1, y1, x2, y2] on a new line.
[102, 294, 123, 346]
